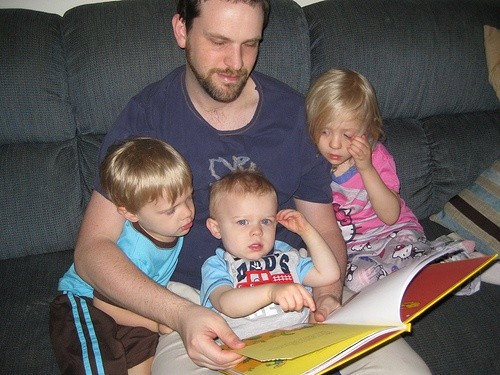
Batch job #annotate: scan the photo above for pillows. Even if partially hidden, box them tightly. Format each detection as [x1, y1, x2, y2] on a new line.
[429, 158, 500, 260]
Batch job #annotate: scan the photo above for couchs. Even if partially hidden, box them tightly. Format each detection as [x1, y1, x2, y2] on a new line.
[0, 0, 500, 375]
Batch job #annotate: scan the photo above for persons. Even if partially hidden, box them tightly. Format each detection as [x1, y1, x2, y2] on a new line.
[48, 136, 196, 375]
[305, 67, 500, 298]
[199, 171, 342, 346]
[74, 0, 433, 375]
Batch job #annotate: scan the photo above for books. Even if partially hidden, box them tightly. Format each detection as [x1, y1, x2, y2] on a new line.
[216, 241, 500, 375]
